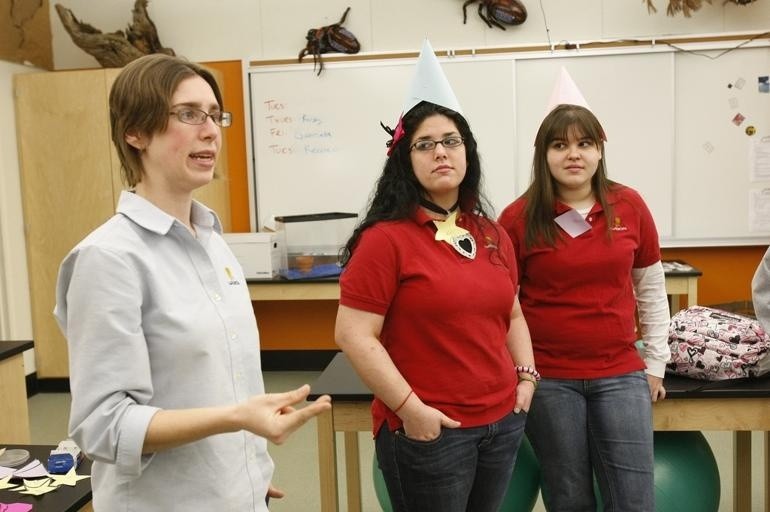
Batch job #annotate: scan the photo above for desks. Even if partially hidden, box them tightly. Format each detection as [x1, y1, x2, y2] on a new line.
[1, 443, 91, 510]
[0, 339, 38, 445]
[243, 256, 704, 321]
[307, 344, 768, 510]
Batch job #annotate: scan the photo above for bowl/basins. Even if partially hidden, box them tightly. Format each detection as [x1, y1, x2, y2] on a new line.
[295, 257, 314, 269]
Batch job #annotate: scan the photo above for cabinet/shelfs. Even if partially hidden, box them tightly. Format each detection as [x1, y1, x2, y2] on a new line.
[11, 69, 230, 387]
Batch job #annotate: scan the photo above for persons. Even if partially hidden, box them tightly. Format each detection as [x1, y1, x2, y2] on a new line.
[54, 54, 334, 512]
[335, 103, 542, 512]
[500, 105, 671, 511]
[751, 244, 770, 336]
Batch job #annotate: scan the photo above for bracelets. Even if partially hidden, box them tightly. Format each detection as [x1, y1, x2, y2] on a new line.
[394, 388, 414, 414]
[520, 375, 538, 388]
[515, 365, 541, 382]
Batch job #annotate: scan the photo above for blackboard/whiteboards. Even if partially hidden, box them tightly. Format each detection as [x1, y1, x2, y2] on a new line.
[246, 38, 770, 257]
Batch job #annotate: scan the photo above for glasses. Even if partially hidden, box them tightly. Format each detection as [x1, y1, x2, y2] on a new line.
[169, 109, 232, 129]
[408, 136, 464, 154]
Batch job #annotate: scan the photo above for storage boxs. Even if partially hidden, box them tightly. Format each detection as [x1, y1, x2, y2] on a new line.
[221, 227, 287, 280]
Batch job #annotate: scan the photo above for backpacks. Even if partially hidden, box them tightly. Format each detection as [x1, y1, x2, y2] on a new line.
[660, 306, 770, 382]
